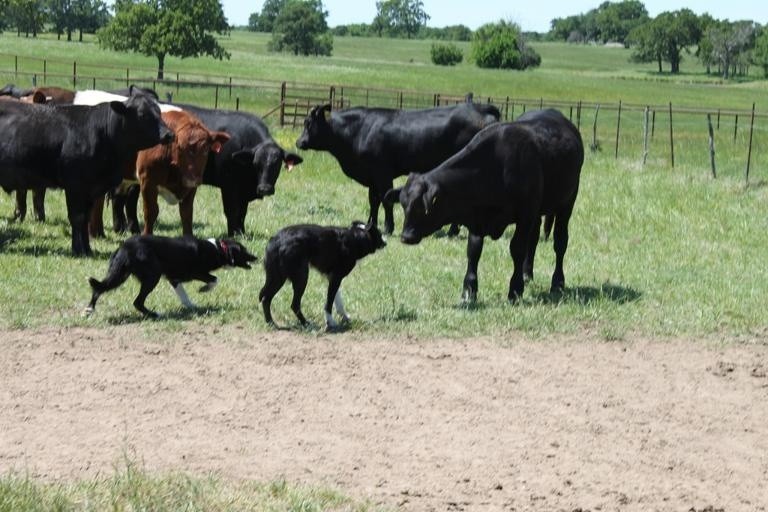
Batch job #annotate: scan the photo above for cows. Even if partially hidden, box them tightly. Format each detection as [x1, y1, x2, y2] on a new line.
[295, 101, 501, 237]
[0, 83, 303, 260]
[383, 108, 585, 310]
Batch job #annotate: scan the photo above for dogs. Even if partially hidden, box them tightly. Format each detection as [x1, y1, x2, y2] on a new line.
[86, 233, 258, 319]
[258, 216, 387, 329]
